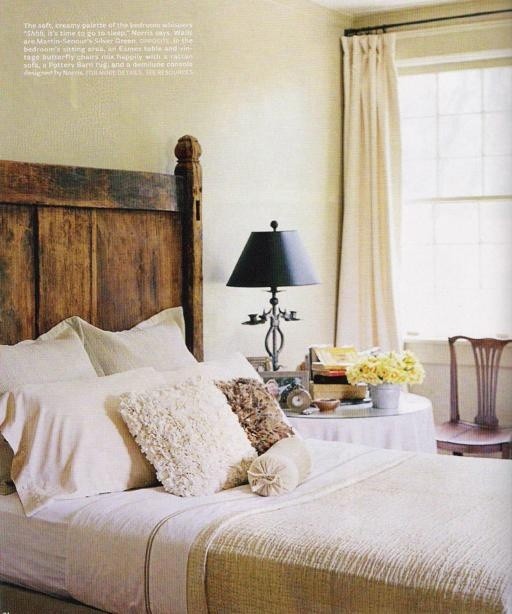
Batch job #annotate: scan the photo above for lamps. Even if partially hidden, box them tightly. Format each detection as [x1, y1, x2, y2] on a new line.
[227, 220, 323, 371]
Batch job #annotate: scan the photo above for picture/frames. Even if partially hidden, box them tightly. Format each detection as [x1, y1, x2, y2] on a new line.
[246, 357, 310, 412]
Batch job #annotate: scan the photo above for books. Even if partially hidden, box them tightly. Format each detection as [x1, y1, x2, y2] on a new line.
[300, 343, 368, 402]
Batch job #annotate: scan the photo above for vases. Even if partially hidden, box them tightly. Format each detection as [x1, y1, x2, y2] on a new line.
[368, 383, 401, 408]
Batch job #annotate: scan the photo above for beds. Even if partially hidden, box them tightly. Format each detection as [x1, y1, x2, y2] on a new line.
[0, 135, 512, 614]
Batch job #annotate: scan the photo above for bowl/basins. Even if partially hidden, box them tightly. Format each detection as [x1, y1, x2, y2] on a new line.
[312, 397, 341, 412]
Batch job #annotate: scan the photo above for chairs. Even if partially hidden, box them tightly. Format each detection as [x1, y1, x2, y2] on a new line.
[436, 336, 512, 459]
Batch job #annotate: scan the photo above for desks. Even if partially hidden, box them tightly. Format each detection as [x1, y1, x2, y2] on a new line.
[286, 388, 436, 452]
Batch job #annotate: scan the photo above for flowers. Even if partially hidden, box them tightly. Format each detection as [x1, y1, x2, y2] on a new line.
[345, 346, 425, 385]
